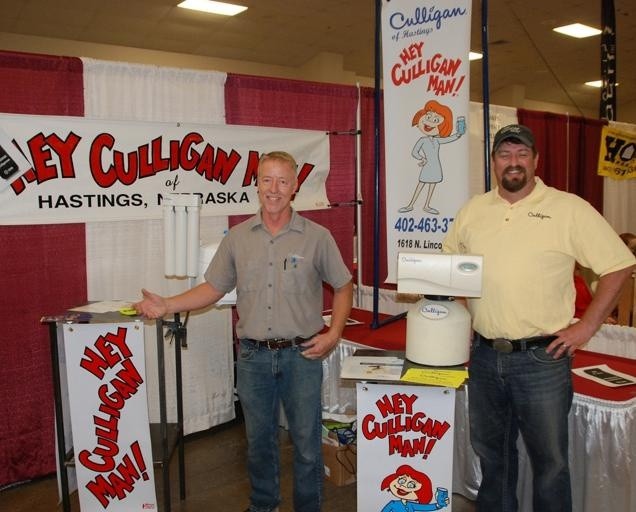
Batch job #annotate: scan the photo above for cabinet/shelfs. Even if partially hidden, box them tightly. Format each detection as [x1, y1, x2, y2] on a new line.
[39, 302, 186, 512]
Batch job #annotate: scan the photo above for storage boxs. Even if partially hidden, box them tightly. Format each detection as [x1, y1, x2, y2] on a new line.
[321, 409, 357, 487]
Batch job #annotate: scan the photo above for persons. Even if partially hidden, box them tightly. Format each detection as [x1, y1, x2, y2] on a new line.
[607, 232, 636, 327]
[398, 99, 466, 215]
[131, 149, 355, 512]
[440, 123, 636, 512]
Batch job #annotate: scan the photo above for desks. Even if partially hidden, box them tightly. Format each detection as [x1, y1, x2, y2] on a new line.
[321, 300, 636, 510]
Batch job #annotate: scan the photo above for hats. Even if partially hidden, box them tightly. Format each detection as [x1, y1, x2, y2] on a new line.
[494, 124, 535, 153]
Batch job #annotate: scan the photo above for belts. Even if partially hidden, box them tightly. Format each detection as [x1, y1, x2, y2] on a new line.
[251, 336, 303, 349]
[479, 336, 555, 353]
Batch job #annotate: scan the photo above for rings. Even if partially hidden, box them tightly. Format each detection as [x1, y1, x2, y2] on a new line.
[562, 343, 569, 348]
